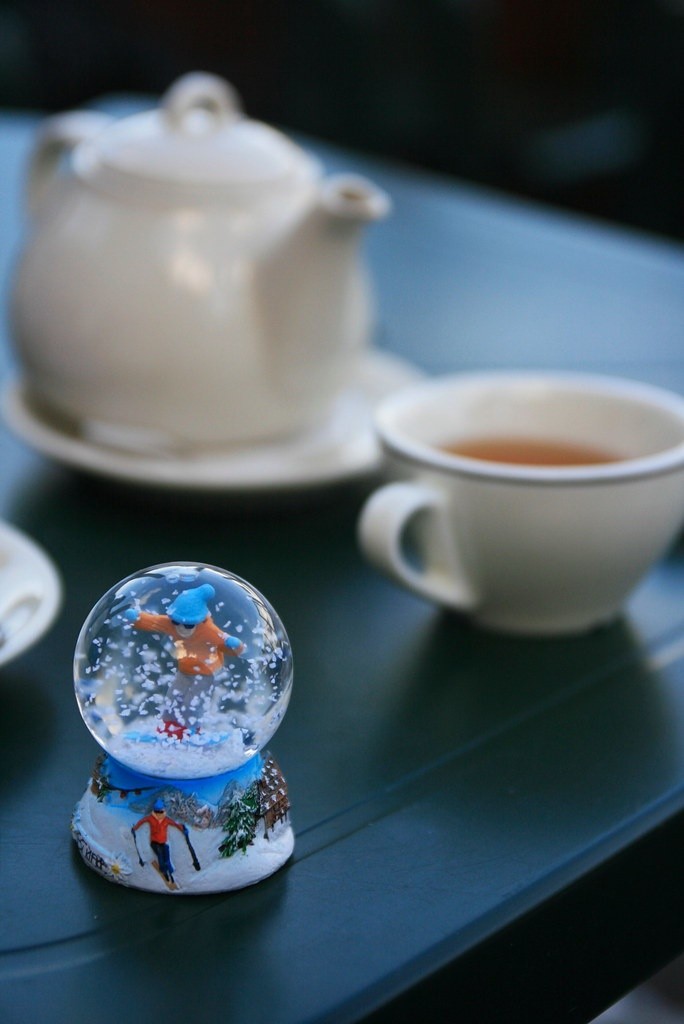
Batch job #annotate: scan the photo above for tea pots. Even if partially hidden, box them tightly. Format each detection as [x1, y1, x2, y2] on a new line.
[14, 73, 387, 456]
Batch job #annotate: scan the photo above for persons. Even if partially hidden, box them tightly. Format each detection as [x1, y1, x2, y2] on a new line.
[134, 800, 189, 883]
[126, 584, 244, 736]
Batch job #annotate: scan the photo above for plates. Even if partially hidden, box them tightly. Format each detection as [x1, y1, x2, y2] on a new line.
[1, 521, 60, 672]
[4, 345, 427, 487]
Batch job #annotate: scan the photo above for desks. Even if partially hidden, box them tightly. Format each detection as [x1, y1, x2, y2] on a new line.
[0, 91, 684, 1024]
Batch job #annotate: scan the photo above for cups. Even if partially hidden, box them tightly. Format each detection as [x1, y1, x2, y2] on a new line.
[359, 373, 684, 634]
